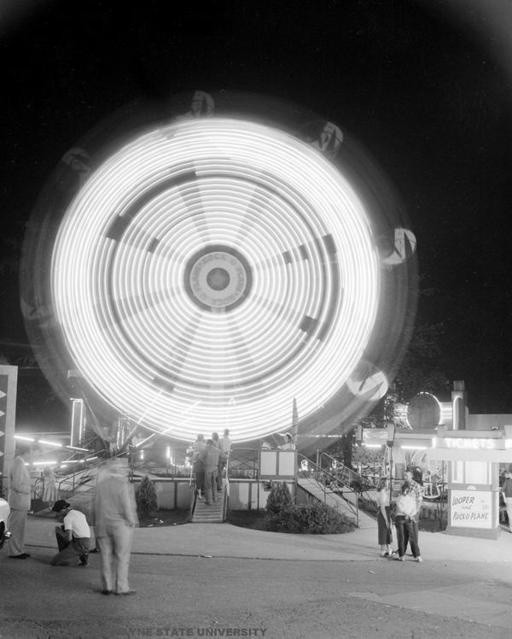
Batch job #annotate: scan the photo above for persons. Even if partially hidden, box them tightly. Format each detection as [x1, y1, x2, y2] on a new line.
[260, 431, 293, 449]
[375, 477, 393, 557]
[397, 469, 423, 555]
[393, 484, 423, 563]
[497, 472, 512, 533]
[88, 462, 137, 596]
[7, 448, 35, 559]
[41, 464, 60, 508]
[50, 500, 92, 567]
[192, 428, 232, 504]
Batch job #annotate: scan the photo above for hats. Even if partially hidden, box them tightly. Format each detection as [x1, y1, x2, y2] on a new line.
[52, 499, 70, 511]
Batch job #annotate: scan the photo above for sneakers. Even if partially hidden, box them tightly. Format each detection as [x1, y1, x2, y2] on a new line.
[80, 550, 88, 566]
